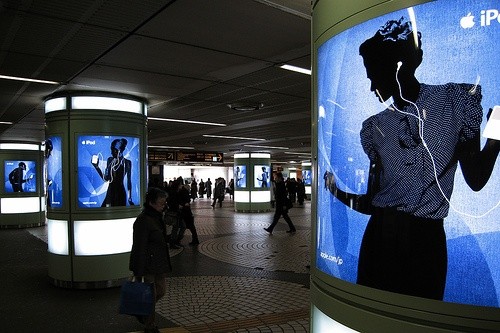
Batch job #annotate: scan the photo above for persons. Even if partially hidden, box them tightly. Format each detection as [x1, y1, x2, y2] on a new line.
[257, 167, 268, 188]
[44, 140, 53, 206]
[264, 173, 297, 233]
[323, 16, 500, 300]
[128, 187, 171, 333]
[283, 178, 305, 206]
[9, 162, 27, 193]
[198, 177, 234, 209]
[91, 138, 132, 207]
[164, 176, 199, 249]
[236, 167, 241, 186]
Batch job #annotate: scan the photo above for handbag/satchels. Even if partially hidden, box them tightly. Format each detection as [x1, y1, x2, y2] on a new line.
[164, 204, 181, 227]
[279, 199, 293, 209]
[117, 276, 154, 316]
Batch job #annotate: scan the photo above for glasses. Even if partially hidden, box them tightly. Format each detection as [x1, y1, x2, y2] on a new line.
[159, 201, 167, 205]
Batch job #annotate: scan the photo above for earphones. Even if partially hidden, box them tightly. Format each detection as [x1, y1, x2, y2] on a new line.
[396, 61, 402, 70]
[118, 150, 121, 153]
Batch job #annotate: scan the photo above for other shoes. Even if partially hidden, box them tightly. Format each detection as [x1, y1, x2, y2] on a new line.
[220, 204, 222, 207]
[176, 242, 184, 247]
[264, 228, 272, 234]
[211, 202, 215, 207]
[144, 326, 160, 333]
[170, 246, 180, 249]
[287, 229, 296, 233]
[189, 239, 199, 245]
